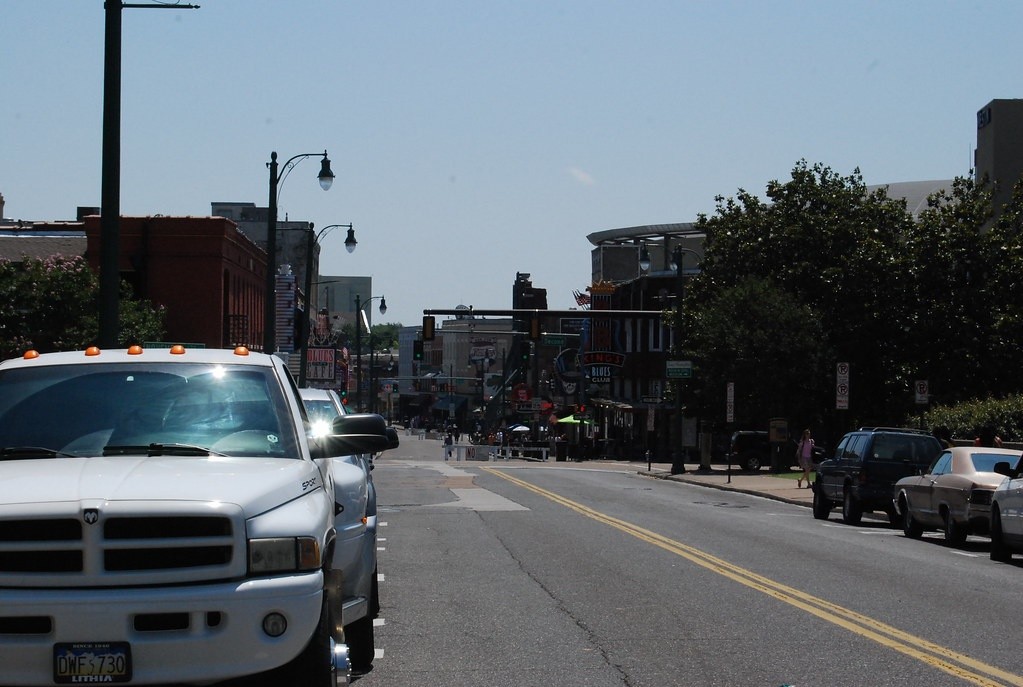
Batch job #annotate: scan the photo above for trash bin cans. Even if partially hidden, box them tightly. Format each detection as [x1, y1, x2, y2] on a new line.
[670, 452, 685, 475]
[555, 441, 567, 462]
[493, 442, 501, 455]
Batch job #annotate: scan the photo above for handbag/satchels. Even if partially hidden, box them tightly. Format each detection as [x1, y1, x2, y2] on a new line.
[811, 439, 815, 459]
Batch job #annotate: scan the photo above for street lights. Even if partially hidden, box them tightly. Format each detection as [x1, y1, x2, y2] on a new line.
[374, 352, 392, 414]
[483, 348, 506, 419]
[354, 293, 388, 414]
[467, 356, 485, 412]
[263, 149, 336, 355]
[637, 241, 687, 475]
[298, 220, 360, 389]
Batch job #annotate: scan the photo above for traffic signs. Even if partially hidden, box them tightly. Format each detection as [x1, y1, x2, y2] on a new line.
[573, 413, 590, 420]
[835, 362, 849, 410]
[665, 360, 693, 379]
[914, 380, 929, 404]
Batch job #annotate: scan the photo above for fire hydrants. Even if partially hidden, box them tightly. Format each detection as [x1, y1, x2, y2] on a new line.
[369, 335, 394, 414]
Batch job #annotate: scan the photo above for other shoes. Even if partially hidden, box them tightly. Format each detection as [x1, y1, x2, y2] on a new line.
[798, 479, 801, 487]
[807, 485, 812, 488]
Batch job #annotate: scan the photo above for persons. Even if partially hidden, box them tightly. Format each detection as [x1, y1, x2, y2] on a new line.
[932, 426, 955, 449]
[455, 424, 464, 444]
[469, 423, 483, 444]
[521, 433, 535, 454]
[798, 429, 815, 488]
[444, 433, 452, 457]
[404, 415, 452, 440]
[547, 433, 560, 442]
[488, 429, 502, 445]
[974, 424, 1002, 448]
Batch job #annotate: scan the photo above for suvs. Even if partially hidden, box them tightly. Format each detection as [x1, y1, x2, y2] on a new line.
[730, 429, 827, 474]
[0, 345, 389, 687]
[811, 427, 943, 528]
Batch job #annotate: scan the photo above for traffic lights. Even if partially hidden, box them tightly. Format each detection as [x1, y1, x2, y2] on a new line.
[413, 340, 424, 361]
[392, 384, 399, 393]
[579, 405, 588, 413]
[340, 381, 348, 405]
[520, 340, 531, 360]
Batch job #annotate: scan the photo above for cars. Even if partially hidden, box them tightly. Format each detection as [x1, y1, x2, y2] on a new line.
[986, 449, 1023, 563]
[891, 444, 1023, 545]
[160, 385, 401, 671]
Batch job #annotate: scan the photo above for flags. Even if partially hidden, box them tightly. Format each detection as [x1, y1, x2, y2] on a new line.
[573, 292, 590, 305]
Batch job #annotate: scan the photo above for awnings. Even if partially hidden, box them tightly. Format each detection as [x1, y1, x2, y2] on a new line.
[433, 397, 466, 410]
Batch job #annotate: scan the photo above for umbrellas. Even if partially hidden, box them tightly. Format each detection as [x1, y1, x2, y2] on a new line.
[509, 424, 521, 428]
[513, 426, 530, 431]
[557, 415, 590, 441]
